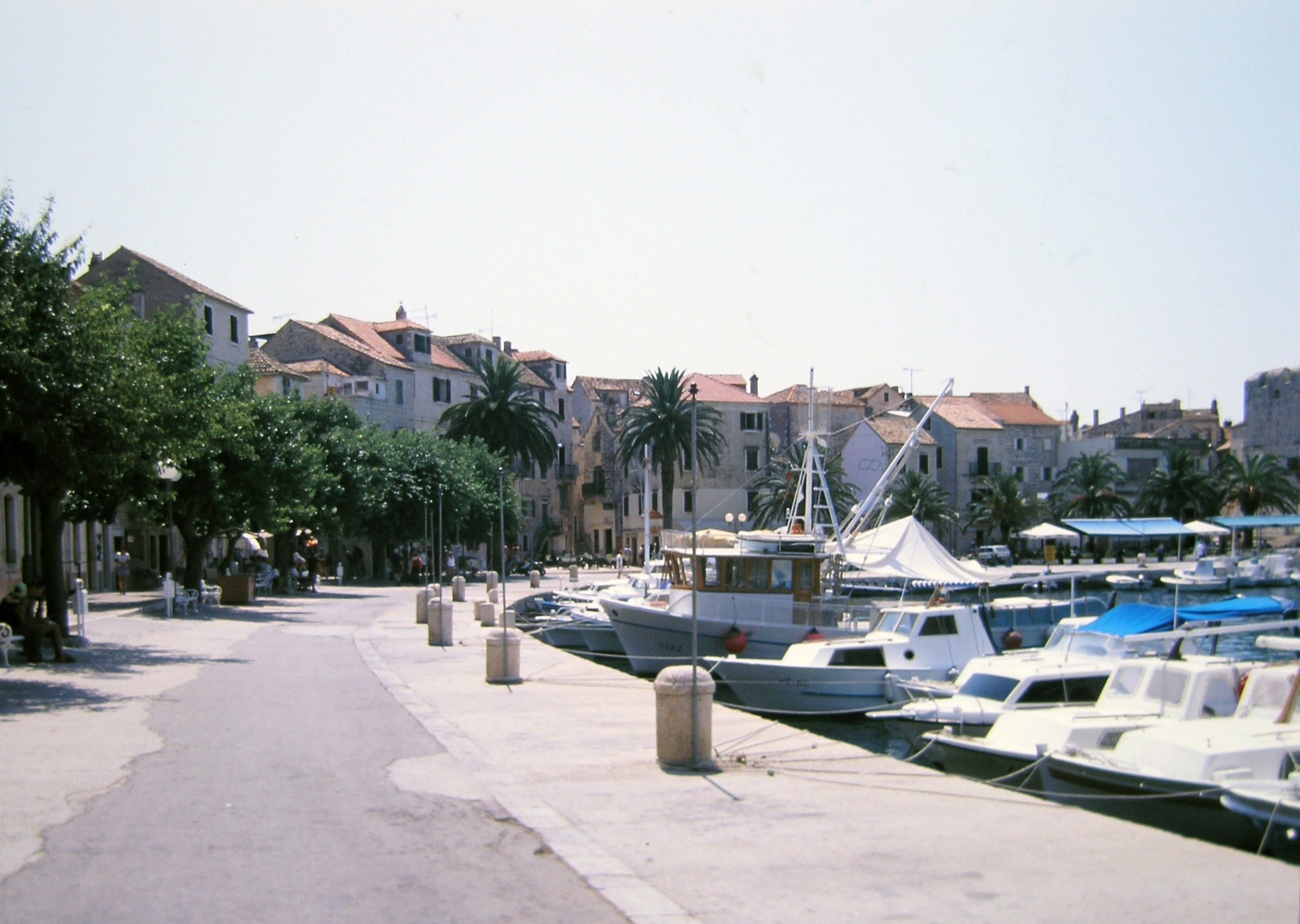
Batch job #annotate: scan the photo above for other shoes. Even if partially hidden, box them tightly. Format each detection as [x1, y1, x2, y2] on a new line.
[54, 654, 75, 664]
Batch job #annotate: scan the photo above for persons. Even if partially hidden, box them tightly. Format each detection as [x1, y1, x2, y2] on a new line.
[639, 544, 645, 566]
[114, 545, 131, 596]
[0, 582, 44, 666]
[208, 547, 329, 594]
[1195, 540, 1206, 561]
[623, 544, 632, 568]
[616, 550, 623, 579]
[20, 577, 74, 664]
[341, 544, 457, 587]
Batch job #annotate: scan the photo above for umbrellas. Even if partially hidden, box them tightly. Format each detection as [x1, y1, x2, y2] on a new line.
[1009, 522, 1077, 572]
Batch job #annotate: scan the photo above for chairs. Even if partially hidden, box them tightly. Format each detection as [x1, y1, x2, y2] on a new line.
[201, 578, 222, 609]
[0, 623, 25, 667]
[256, 568, 280, 595]
[162, 578, 199, 615]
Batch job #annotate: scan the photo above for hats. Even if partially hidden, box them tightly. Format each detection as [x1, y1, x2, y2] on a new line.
[11, 582, 26, 597]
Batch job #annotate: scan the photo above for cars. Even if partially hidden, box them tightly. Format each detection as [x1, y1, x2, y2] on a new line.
[975, 545, 1010, 559]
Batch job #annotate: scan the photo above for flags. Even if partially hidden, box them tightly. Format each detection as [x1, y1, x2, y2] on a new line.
[1182, 521, 1231, 559]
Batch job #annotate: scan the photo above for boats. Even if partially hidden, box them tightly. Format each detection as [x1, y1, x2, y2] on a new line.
[530, 366, 1300, 839]
[1104, 573, 1152, 592]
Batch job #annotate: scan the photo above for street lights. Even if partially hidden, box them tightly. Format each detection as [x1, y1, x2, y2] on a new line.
[155, 459, 182, 581]
